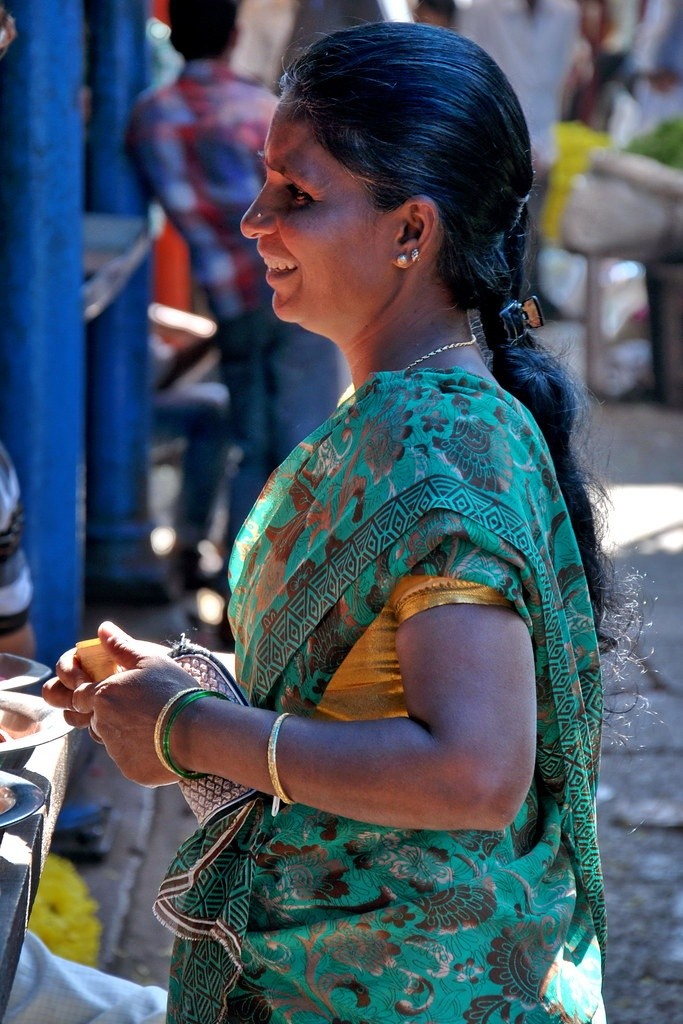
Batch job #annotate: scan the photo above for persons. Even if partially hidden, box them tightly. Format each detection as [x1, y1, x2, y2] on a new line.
[123, 0, 683, 645]
[41, 20, 619, 1024]
[0, 441, 35, 660]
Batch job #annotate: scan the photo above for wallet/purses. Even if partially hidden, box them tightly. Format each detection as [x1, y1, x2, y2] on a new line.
[152, 645, 264, 833]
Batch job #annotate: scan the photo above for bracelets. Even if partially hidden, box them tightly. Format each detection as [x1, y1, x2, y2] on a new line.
[268, 708, 296, 809]
[154, 687, 232, 782]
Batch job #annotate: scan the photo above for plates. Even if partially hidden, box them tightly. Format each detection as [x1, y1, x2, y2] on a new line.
[0, 770, 45, 829]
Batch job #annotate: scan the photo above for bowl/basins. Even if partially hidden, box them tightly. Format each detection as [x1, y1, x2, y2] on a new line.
[0, 691, 76, 769]
[0, 652, 53, 692]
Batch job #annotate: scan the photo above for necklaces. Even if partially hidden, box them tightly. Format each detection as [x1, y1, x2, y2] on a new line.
[404, 334, 476, 370]
